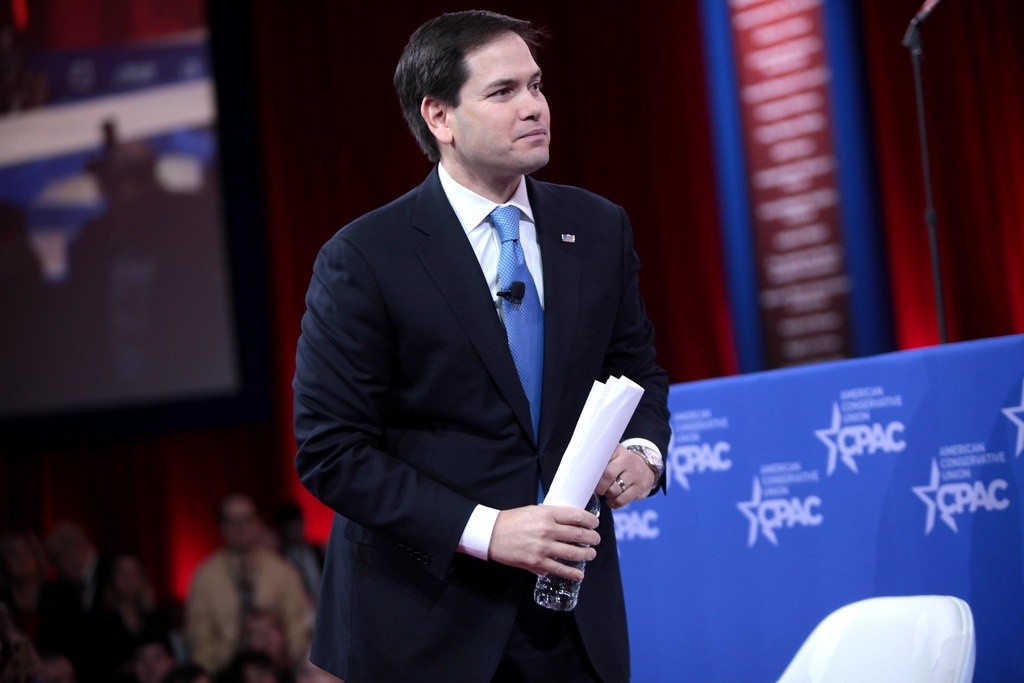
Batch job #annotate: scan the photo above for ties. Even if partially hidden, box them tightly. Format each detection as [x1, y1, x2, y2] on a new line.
[489, 205, 545, 507]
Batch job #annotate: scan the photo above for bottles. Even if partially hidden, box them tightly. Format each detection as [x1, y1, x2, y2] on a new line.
[533, 506, 600, 611]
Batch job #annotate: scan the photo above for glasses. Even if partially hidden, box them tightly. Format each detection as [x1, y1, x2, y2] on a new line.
[223, 512, 259, 525]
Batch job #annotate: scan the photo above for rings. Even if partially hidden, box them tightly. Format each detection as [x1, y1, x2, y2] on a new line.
[616, 477, 626, 493]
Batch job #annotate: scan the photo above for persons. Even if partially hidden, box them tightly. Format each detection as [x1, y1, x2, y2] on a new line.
[6, 492, 316, 682]
[292, 10, 673, 681]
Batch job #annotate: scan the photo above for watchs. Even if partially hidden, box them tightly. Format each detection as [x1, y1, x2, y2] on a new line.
[627, 445, 664, 489]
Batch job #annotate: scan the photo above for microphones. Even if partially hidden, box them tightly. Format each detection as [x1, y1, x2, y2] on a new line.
[510, 281, 526, 305]
